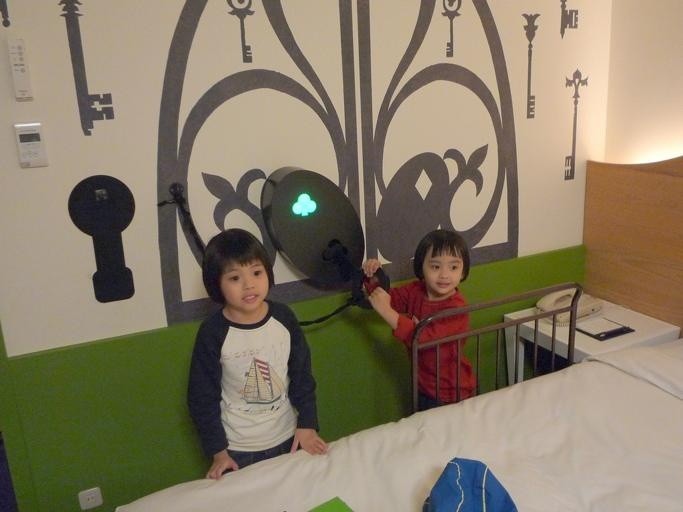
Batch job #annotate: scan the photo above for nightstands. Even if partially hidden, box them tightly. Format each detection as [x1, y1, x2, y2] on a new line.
[505, 291, 680, 390]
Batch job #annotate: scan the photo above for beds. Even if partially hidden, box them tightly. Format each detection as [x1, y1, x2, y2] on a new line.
[114, 337, 683, 511]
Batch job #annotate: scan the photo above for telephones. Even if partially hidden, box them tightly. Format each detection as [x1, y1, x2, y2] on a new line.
[534, 288, 602, 326]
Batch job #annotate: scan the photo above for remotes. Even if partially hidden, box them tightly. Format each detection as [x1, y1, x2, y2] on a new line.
[7, 38, 33, 102]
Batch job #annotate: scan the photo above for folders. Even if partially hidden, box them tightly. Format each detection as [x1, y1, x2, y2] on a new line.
[574, 315, 635, 342]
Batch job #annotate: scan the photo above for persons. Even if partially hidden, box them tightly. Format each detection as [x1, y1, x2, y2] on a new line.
[189, 228, 330, 481]
[363, 229, 480, 415]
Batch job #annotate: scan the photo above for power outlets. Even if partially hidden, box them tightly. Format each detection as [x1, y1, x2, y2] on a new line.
[76, 485, 103, 511]
